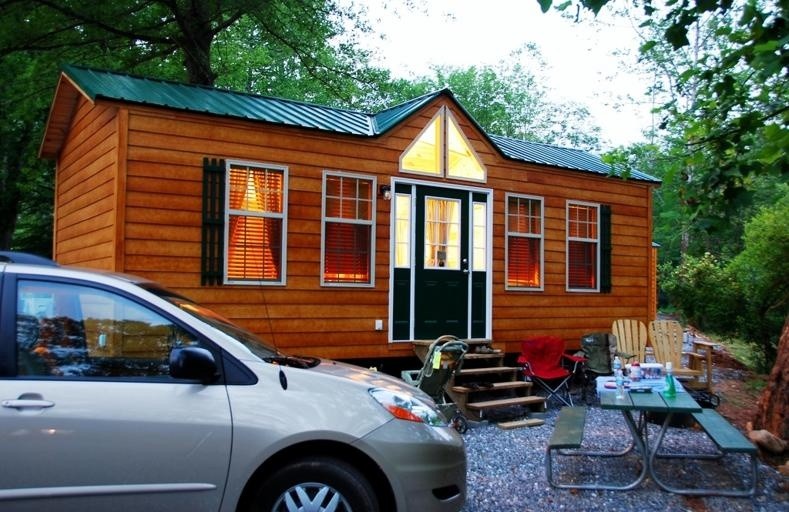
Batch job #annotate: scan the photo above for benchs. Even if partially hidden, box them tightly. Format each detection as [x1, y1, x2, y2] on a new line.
[545, 405, 587, 490]
[692, 409, 759, 498]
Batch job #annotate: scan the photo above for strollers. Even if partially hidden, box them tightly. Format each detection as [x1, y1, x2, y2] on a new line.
[399, 334, 469, 435]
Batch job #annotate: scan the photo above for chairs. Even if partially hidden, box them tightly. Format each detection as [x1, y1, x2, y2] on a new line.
[517, 319, 706, 413]
[16, 315, 88, 376]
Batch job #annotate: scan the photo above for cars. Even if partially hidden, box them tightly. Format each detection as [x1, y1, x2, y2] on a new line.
[0, 248, 467, 512]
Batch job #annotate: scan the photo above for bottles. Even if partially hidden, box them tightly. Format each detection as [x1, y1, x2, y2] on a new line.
[663, 362, 676, 398]
[612, 356, 625, 402]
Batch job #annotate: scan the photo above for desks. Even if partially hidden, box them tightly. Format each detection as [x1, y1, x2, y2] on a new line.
[600, 375, 703, 492]
